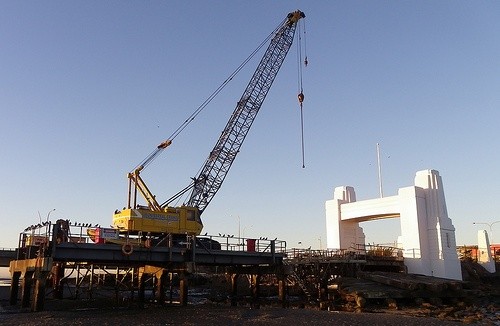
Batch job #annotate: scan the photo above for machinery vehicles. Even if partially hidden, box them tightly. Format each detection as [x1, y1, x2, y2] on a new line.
[112, 8, 307, 249]
